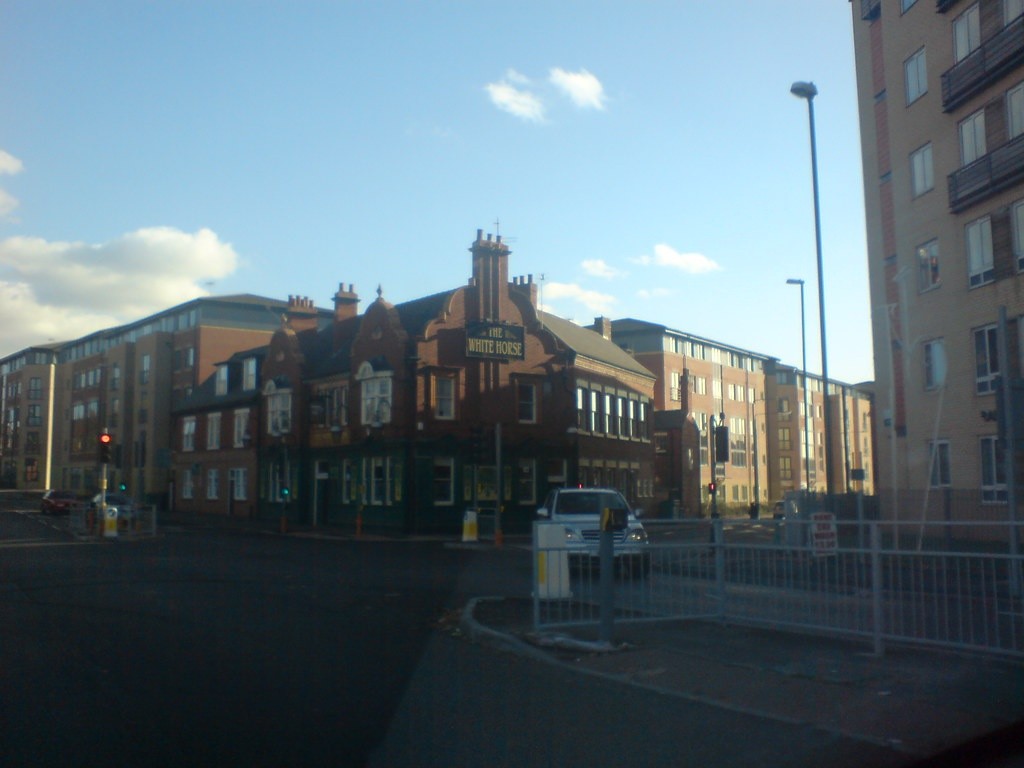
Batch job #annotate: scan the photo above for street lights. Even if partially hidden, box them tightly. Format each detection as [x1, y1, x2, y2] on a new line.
[792, 80, 834, 516]
[786, 277, 811, 518]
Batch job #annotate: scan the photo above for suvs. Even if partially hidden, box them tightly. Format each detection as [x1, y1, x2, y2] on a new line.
[537, 486, 650, 583]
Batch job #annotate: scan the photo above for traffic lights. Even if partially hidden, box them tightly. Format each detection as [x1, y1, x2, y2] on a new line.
[708, 482, 716, 493]
[99, 433, 113, 465]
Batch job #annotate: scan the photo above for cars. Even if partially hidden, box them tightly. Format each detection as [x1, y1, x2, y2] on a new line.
[39, 487, 84, 517]
[90, 492, 137, 528]
[773, 500, 798, 519]
